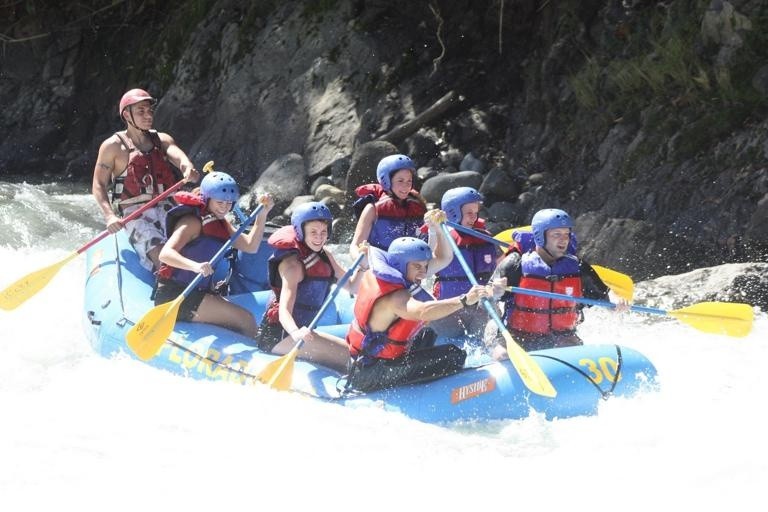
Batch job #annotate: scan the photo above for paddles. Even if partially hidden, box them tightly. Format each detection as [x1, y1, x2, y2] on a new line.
[494, 225, 533, 254]
[505, 286, 754, 336]
[256, 249, 365, 389]
[427, 203, 556, 398]
[126, 203, 262, 362]
[446, 220, 633, 297]
[0, 178, 188, 310]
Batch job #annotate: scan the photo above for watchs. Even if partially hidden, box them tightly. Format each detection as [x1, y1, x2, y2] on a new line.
[460, 294, 468, 307]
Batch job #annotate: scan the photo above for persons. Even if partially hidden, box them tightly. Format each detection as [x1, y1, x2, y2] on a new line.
[347, 206, 493, 392]
[254, 202, 369, 372]
[423, 185, 504, 337]
[154, 170, 275, 339]
[350, 152, 431, 271]
[90, 86, 202, 271]
[483, 206, 632, 353]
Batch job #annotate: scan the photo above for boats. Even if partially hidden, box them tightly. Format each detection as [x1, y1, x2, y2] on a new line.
[83, 225, 657, 421]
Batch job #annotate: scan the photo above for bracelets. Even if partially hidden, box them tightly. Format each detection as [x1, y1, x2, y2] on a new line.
[427, 228, 437, 237]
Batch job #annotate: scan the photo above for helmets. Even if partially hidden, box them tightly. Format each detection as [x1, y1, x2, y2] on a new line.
[291, 202, 332, 243]
[200, 172, 239, 212]
[531, 209, 572, 247]
[119, 89, 153, 121]
[388, 237, 433, 278]
[441, 187, 481, 223]
[377, 154, 416, 189]
